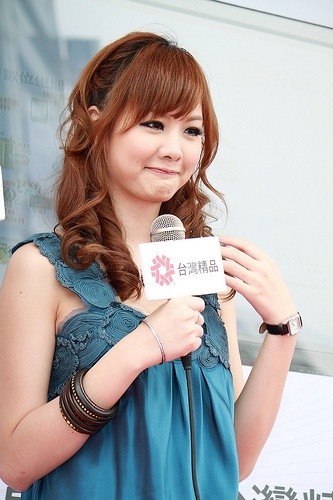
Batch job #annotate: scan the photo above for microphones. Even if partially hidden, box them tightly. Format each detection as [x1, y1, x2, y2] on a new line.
[150, 215, 193, 370]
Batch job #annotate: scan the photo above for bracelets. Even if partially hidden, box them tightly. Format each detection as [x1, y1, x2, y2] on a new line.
[58, 368, 118, 435]
[139, 320, 165, 364]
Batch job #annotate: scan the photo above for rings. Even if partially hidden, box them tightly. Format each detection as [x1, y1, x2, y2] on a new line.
[196, 311, 200, 324]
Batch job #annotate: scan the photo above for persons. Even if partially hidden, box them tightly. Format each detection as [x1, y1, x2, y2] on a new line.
[0, 30, 305, 500]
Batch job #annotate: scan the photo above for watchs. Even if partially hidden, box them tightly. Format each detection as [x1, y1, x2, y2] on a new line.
[258, 313, 303, 335]
